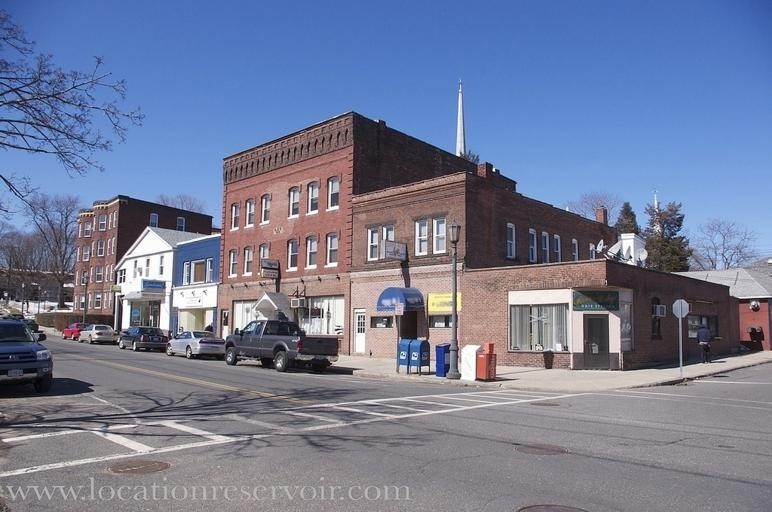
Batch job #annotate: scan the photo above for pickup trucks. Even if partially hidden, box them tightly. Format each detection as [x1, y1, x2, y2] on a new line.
[225, 319, 339, 374]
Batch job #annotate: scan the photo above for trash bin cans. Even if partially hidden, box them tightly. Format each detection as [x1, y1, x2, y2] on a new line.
[396, 339, 430, 368]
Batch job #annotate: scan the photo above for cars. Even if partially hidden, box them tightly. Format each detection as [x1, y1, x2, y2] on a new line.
[77, 324, 117, 346]
[119, 325, 170, 353]
[61, 322, 89, 340]
[166, 330, 227, 361]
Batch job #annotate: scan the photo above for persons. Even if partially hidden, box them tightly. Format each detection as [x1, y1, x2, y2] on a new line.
[696, 324, 714, 365]
[204, 322, 214, 332]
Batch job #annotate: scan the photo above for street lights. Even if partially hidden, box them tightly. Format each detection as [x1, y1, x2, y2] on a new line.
[82, 275, 90, 323]
[444, 218, 462, 379]
[21, 281, 25, 315]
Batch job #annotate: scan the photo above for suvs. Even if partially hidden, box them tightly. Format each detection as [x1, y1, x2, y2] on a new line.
[1, 313, 54, 393]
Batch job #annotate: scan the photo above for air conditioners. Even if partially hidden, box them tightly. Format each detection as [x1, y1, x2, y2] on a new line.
[290, 297, 306, 309]
[650, 303, 667, 318]
